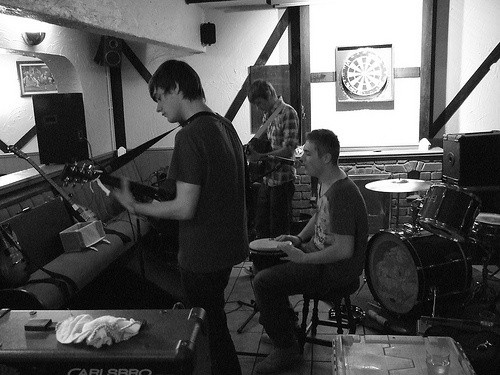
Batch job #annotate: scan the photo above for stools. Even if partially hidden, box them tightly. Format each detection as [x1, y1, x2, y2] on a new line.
[299, 277, 360, 354]
[237, 265, 264, 334]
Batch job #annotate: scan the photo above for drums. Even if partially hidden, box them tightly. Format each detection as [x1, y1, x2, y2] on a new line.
[473, 213, 500, 260]
[416, 184, 482, 243]
[249, 238, 292, 276]
[363, 230, 473, 316]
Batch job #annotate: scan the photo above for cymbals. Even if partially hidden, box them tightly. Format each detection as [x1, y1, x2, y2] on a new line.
[364, 177, 431, 193]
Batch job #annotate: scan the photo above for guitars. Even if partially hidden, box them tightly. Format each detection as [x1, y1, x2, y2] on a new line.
[61, 161, 158, 202]
[244, 142, 301, 168]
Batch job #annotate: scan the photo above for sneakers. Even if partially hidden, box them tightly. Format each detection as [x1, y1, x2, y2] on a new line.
[261, 334, 272, 344]
[255, 347, 303, 375]
[245, 267, 253, 274]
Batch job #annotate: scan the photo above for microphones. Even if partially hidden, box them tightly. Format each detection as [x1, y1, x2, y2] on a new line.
[266, 154, 301, 169]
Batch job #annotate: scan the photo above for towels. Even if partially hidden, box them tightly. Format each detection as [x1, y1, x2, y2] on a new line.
[55, 314, 142, 348]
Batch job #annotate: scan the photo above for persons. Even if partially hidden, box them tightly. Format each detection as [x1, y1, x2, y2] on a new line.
[23, 67, 54, 88]
[111, 60, 250, 375]
[243, 79, 298, 277]
[251, 130, 369, 375]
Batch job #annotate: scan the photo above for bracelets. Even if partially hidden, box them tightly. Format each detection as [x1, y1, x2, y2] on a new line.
[296, 235, 303, 242]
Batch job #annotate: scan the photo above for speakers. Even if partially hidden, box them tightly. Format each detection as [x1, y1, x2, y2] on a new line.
[32, 92, 89, 165]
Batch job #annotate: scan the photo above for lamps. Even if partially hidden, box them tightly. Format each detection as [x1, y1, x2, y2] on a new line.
[22, 23, 46, 46]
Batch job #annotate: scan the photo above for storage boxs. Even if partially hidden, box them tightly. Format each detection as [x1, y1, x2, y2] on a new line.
[332, 333, 477, 375]
[59, 220, 106, 253]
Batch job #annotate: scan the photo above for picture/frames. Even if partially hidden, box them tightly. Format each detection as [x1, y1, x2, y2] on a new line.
[16, 61, 58, 97]
